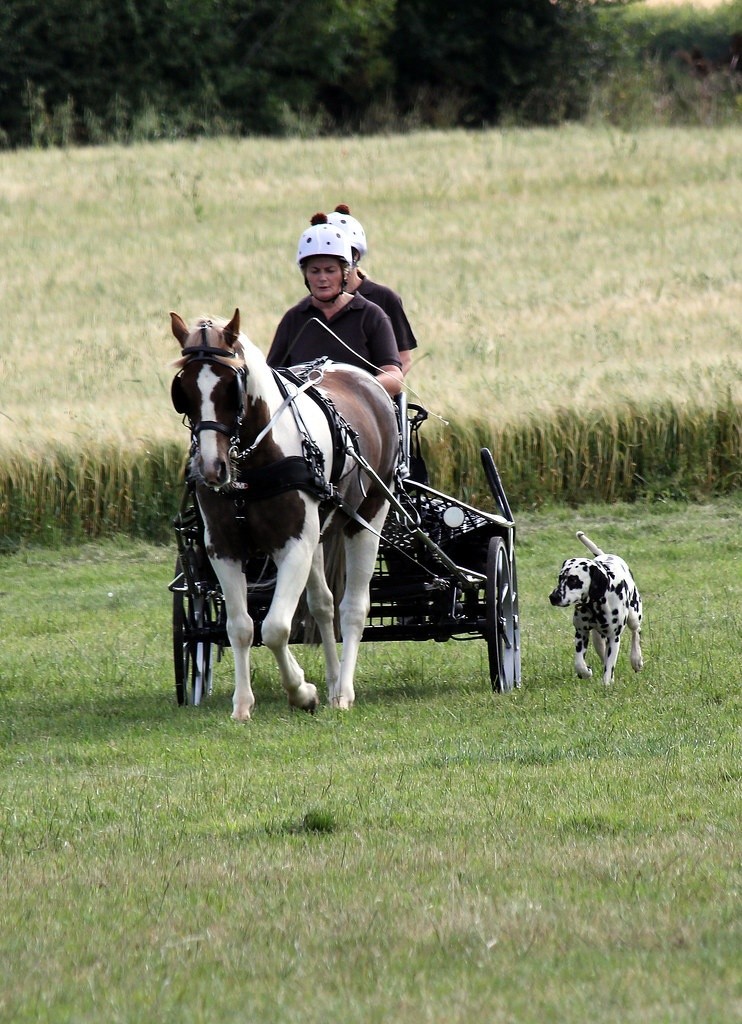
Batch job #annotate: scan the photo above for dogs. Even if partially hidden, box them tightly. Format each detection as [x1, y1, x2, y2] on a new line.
[549, 532, 644, 687]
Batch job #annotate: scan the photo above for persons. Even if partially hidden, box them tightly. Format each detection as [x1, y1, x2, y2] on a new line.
[326, 205, 419, 376]
[264, 211, 404, 398]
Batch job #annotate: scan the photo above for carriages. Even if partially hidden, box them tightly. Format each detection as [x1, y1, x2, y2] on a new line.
[153, 306, 526, 726]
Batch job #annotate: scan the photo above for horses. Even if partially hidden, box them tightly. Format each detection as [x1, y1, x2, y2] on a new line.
[169, 308, 399, 722]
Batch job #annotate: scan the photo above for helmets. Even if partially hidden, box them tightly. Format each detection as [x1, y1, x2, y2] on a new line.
[326, 205, 368, 260]
[296, 214, 353, 276]
